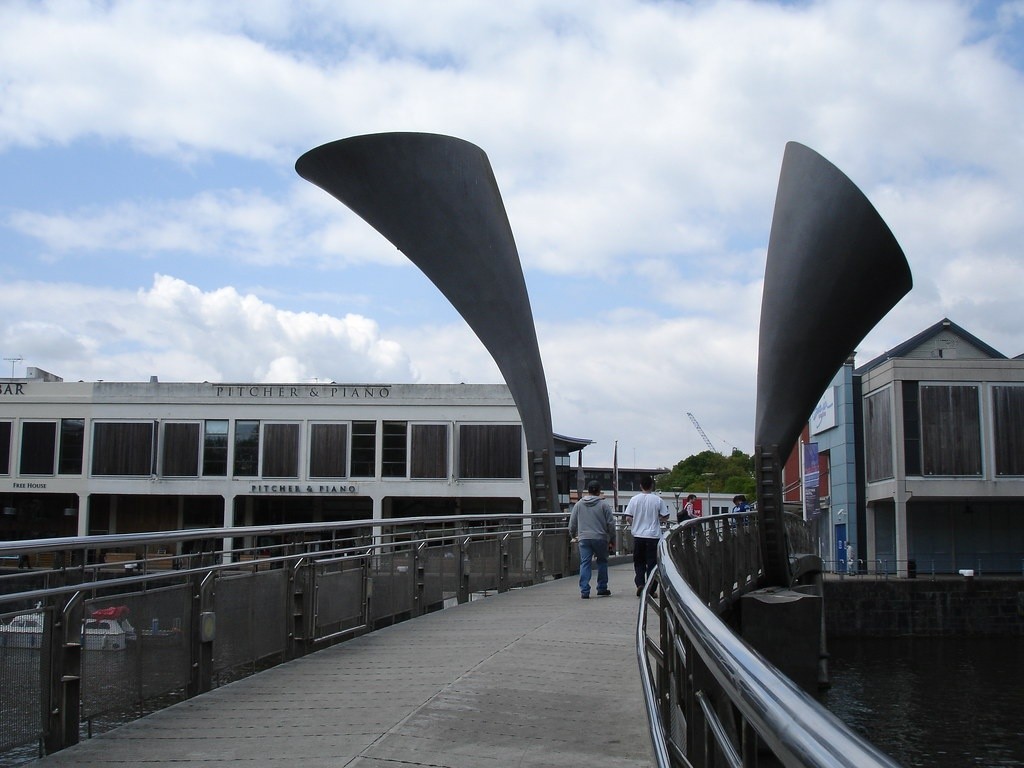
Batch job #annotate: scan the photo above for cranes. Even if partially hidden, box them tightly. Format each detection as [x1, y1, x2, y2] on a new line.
[685, 411, 716, 453]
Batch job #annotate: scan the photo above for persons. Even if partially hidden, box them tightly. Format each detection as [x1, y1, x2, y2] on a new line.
[846, 541, 855, 573]
[684, 493, 699, 520]
[731, 496, 751, 526]
[569, 480, 615, 599]
[624, 476, 670, 598]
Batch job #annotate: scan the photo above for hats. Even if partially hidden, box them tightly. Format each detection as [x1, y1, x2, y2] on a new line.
[588, 480, 604, 492]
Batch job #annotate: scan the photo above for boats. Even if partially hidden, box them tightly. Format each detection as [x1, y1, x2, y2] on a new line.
[1, 614, 126, 652]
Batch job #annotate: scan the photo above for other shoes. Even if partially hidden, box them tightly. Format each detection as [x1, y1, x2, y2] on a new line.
[652, 592, 658, 599]
[596, 590, 613, 598]
[581, 594, 590, 599]
[636, 586, 644, 597]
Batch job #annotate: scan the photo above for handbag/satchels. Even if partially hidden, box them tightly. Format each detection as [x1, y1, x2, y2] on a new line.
[676, 504, 695, 523]
